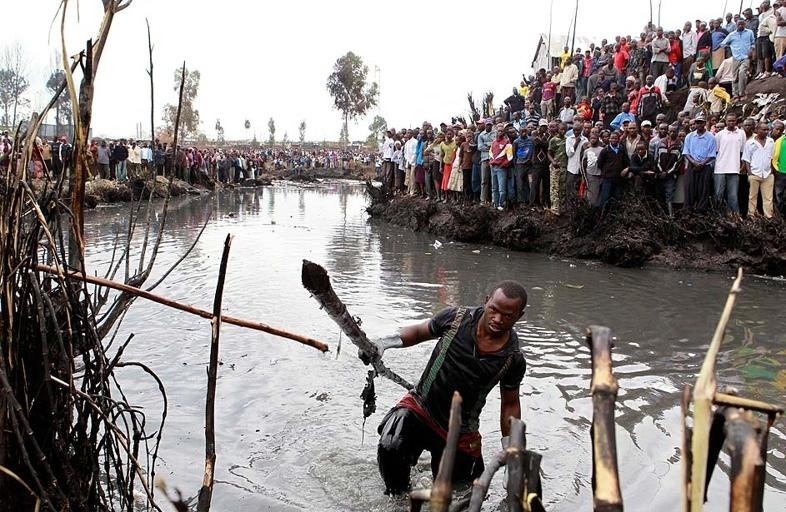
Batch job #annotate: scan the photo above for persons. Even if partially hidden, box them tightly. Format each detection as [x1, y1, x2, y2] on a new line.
[1, 129, 230, 187]
[229, 144, 383, 184]
[365, 281, 526, 493]
[375, 0, 785, 221]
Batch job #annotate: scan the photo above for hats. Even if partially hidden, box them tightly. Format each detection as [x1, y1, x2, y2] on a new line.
[539, 119, 549, 126]
[693, 116, 705, 121]
[504, 123, 513, 129]
[476, 119, 484, 124]
[641, 120, 651, 126]
[485, 119, 493, 126]
